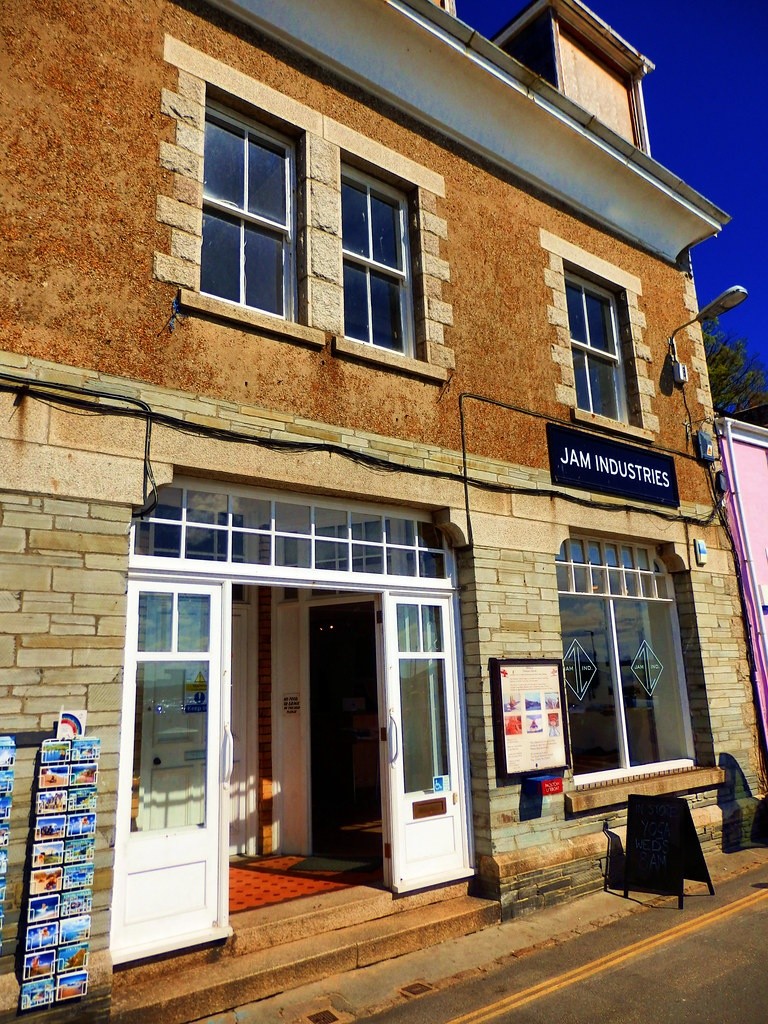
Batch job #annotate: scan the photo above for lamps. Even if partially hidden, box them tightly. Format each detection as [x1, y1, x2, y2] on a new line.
[668, 284, 747, 383]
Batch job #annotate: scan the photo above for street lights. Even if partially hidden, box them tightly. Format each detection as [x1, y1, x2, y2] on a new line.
[584, 629, 600, 671]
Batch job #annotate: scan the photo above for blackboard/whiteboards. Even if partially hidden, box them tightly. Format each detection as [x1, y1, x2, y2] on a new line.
[624, 793, 712, 895]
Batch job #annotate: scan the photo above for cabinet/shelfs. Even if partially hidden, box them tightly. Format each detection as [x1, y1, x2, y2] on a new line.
[568, 706, 657, 766]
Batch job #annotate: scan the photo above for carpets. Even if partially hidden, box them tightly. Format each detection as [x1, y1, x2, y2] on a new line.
[228, 852, 383, 916]
